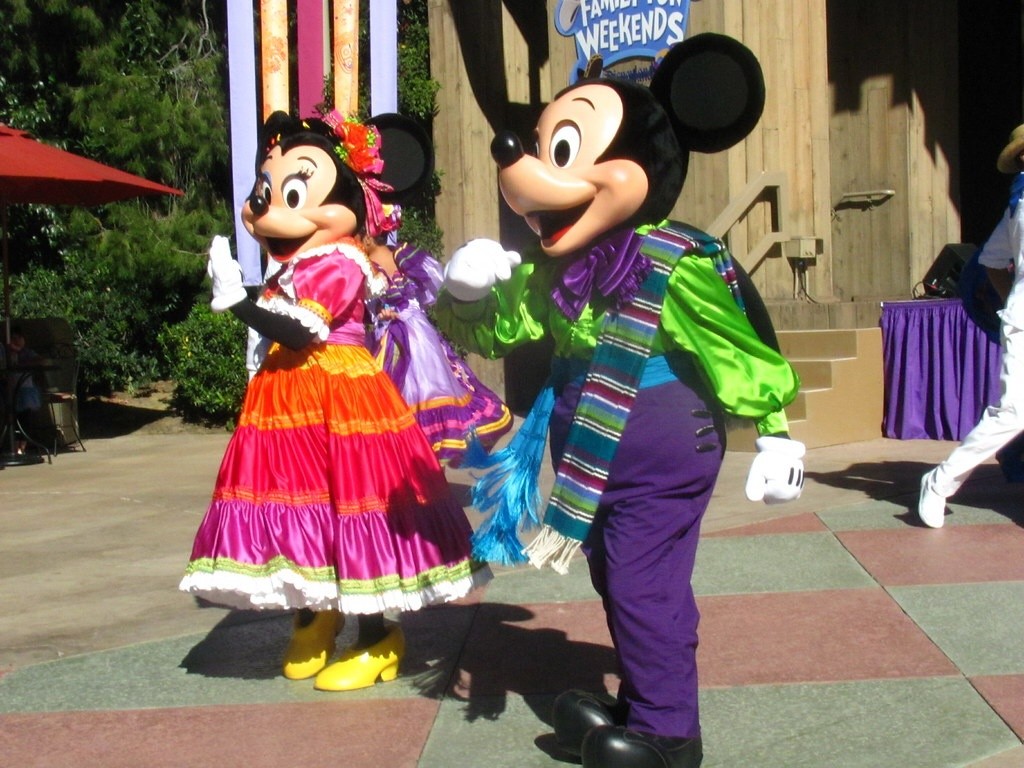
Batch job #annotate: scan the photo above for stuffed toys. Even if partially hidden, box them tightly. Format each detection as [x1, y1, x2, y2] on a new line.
[443, 34, 805, 768]
[179, 112, 514, 692]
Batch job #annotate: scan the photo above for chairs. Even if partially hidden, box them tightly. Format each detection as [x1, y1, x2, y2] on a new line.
[0, 338, 88, 465]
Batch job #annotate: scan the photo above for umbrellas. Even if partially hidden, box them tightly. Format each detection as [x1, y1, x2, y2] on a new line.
[0, 121, 185, 344]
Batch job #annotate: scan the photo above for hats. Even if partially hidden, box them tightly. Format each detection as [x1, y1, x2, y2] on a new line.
[997, 124, 1024, 173]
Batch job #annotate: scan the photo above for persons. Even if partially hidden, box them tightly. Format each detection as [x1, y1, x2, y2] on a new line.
[917, 127, 1023, 527]
[0, 323, 48, 456]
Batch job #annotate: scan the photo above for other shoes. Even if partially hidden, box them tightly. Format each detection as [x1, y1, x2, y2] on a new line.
[918, 472, 946, 528]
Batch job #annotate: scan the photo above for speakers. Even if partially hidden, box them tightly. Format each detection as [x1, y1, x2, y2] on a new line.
[921, 243, 984, 297]
